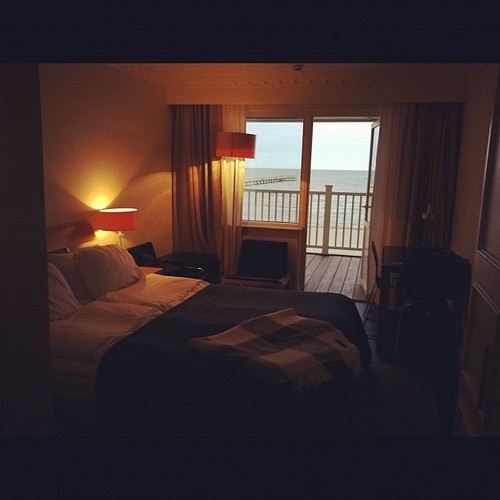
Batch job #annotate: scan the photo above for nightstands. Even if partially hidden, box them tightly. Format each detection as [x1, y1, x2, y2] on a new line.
[140, 266, 163, 276]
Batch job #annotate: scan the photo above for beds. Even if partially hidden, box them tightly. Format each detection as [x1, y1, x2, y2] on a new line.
[46, 219, 375, 432]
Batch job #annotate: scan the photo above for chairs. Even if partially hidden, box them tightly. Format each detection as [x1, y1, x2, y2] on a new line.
[223, 239, 291, 288]
[129, 242, 207, 278]
[363, 241, 405, 326]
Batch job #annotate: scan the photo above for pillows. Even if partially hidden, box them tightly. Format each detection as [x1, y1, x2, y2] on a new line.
[47, 262, 82, 320]
[76, 244, 144, 299]
[47, 252, 93, 306]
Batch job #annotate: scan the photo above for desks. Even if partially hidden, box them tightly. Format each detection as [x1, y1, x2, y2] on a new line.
[378, 244, 451, 362]
[158, 252, 219, 281]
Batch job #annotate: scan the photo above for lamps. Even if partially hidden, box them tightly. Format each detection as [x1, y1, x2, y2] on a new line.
[216, 131, 255, 279]
[99, 207, 138, 247]
